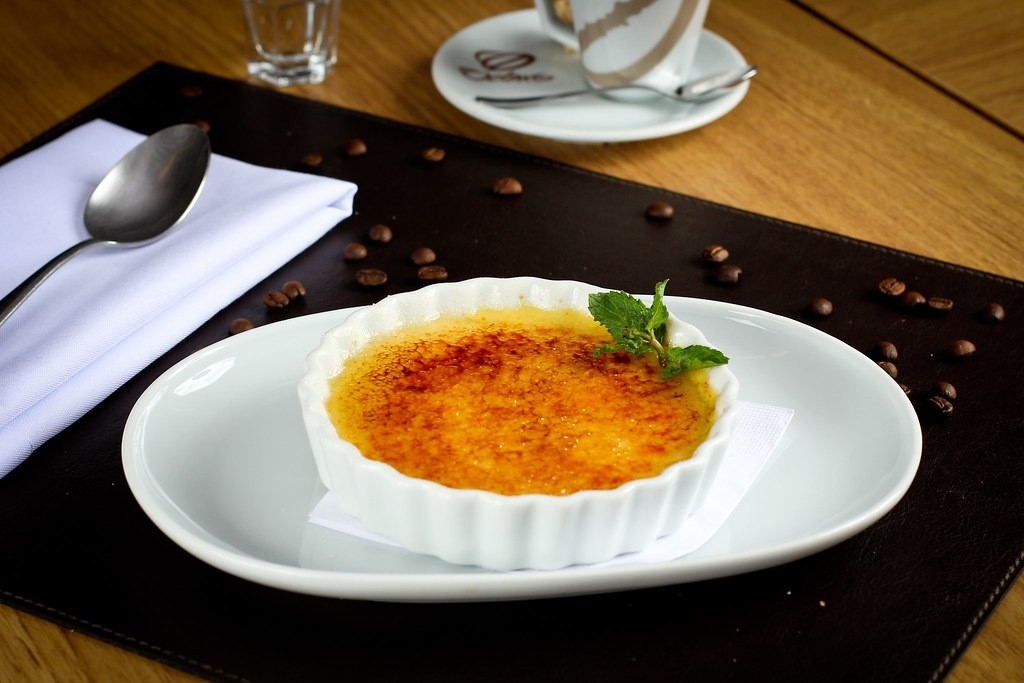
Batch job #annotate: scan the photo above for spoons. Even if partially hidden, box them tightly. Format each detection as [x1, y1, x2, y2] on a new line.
[0, 123, 212, 327]
[474, 62, 760, 107]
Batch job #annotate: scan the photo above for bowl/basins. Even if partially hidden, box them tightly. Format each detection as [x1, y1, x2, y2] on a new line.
[297, 273, 744, 569]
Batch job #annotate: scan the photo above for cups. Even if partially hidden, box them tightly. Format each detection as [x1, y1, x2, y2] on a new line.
[237, 0, 340, 88]
[535, 0, 710, 103]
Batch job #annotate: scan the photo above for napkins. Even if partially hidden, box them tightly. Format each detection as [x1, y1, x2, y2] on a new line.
[0, 116, 364, 476]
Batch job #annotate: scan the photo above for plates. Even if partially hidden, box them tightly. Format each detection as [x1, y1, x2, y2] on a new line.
[429, 7, 751, 146]
[121, 292, 925, 604]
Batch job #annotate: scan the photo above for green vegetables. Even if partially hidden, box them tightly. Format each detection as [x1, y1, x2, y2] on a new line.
[588, 278, 730, 379]
